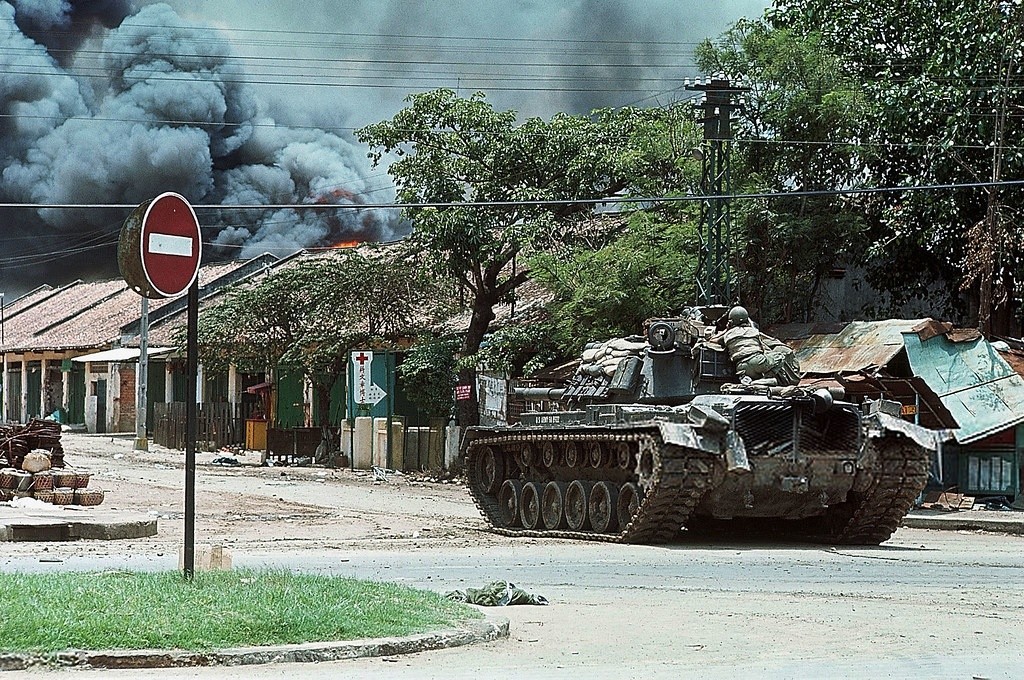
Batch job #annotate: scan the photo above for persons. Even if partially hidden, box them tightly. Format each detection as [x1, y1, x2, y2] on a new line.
[724, 305, 785, 386]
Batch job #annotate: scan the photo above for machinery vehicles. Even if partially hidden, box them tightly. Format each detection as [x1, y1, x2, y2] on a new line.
[455, 275, 939, 547]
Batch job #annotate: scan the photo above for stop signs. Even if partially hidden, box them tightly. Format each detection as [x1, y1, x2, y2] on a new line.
[139, 191, 203, 299]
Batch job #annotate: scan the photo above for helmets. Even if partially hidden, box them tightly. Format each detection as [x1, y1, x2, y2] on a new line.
[727, 306, 749, 325]
